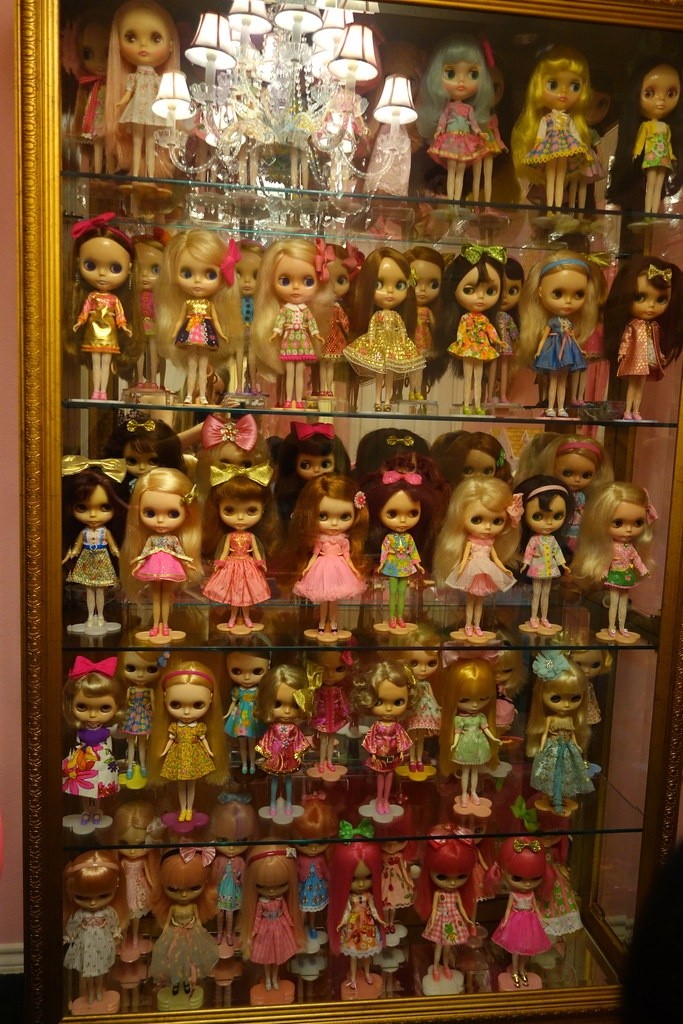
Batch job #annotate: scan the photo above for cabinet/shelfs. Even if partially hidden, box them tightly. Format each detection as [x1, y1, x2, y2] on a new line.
[16, 1, 683, 1024]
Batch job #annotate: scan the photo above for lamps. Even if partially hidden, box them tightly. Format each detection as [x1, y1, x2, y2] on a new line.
[152, 0, 419, 243]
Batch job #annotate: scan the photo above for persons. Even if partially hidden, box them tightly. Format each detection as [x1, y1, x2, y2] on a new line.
[61, 418, 652, 1004]
[66, 0, 681, 422]
